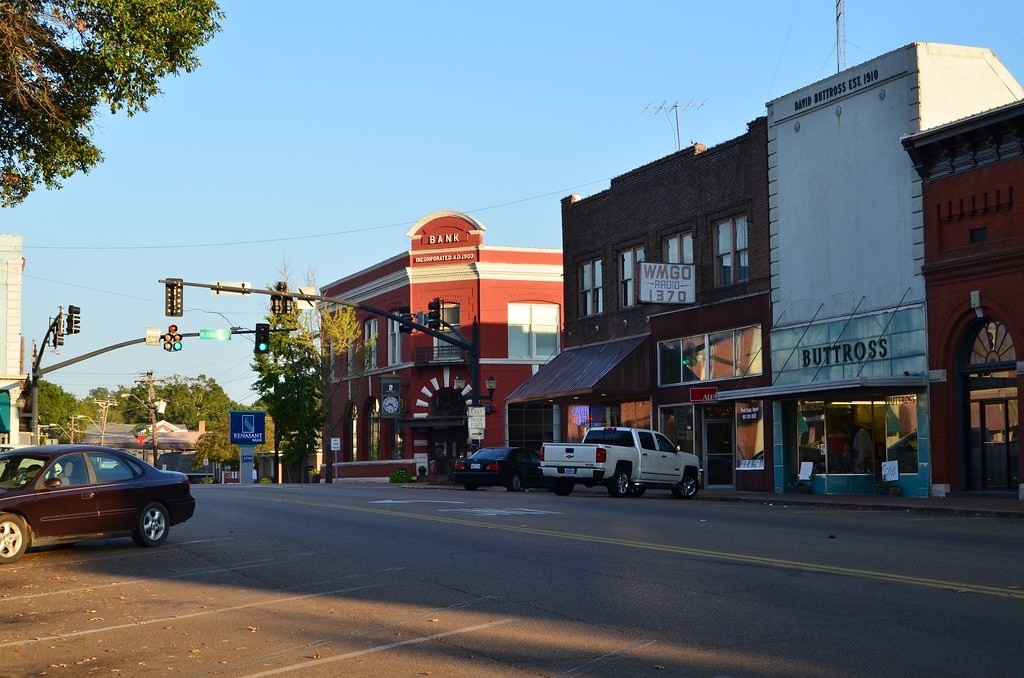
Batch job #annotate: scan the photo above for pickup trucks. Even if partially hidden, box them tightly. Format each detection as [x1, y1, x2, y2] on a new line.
[537, 426, 704, 499]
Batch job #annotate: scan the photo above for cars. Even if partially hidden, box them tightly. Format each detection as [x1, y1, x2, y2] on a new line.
[452, 446, 555, 492]
[0, 444, 196, 566]
[886, 425, 992, 474]
[736, 445, 826, 494]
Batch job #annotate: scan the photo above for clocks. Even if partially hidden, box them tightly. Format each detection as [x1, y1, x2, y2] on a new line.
[383, 396, 398, 413]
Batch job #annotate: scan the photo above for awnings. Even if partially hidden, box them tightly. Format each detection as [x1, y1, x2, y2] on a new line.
[504, 332, 651, 404]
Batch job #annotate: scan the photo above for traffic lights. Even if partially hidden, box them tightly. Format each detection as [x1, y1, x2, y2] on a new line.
[255, 323, 270, 354]
[53, 319, 64, 349]
[164, 333, 173, 352]
[169, 325, 178, 334]
[428, 297, 441, 330]
[173, 333, 182, 351]
[69, 305, 81, 314]
[399, 304, 412, 334]
[66, 315, 81, 335]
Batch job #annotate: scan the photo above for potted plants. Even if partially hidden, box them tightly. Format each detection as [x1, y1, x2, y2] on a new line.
[308, 469, 321, 483]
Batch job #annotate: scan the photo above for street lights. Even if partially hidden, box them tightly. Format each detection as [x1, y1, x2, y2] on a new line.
[121, 393, 158, 468]
[76, 414, 104, 447]
[49, 423, 74, 444]
